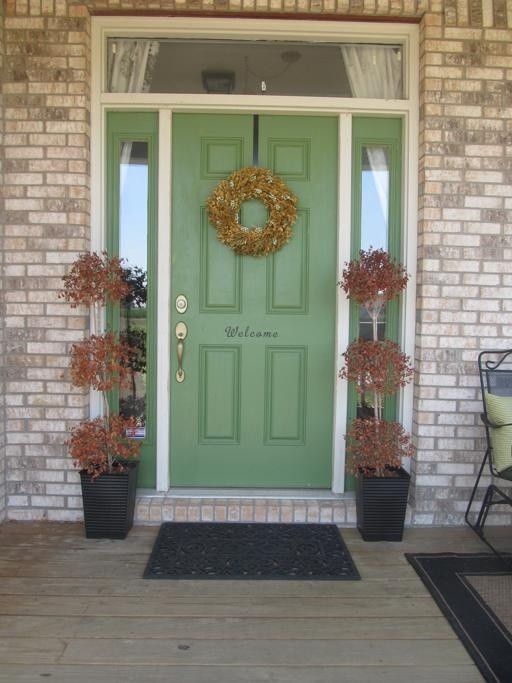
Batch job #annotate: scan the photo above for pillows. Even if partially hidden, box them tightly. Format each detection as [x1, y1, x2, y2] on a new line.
[479, 392, 512, 472]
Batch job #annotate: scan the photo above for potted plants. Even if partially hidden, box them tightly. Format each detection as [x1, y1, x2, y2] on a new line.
[62, 248, 139, 538]
[338, 252, 417, 540]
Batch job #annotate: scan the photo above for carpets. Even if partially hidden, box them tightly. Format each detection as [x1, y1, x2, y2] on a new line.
[143, 518, 361, 583]
[407, 549, 511, 683]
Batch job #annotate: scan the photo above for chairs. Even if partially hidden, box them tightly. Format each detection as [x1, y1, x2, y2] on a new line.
[468, 348, 512, 562]
